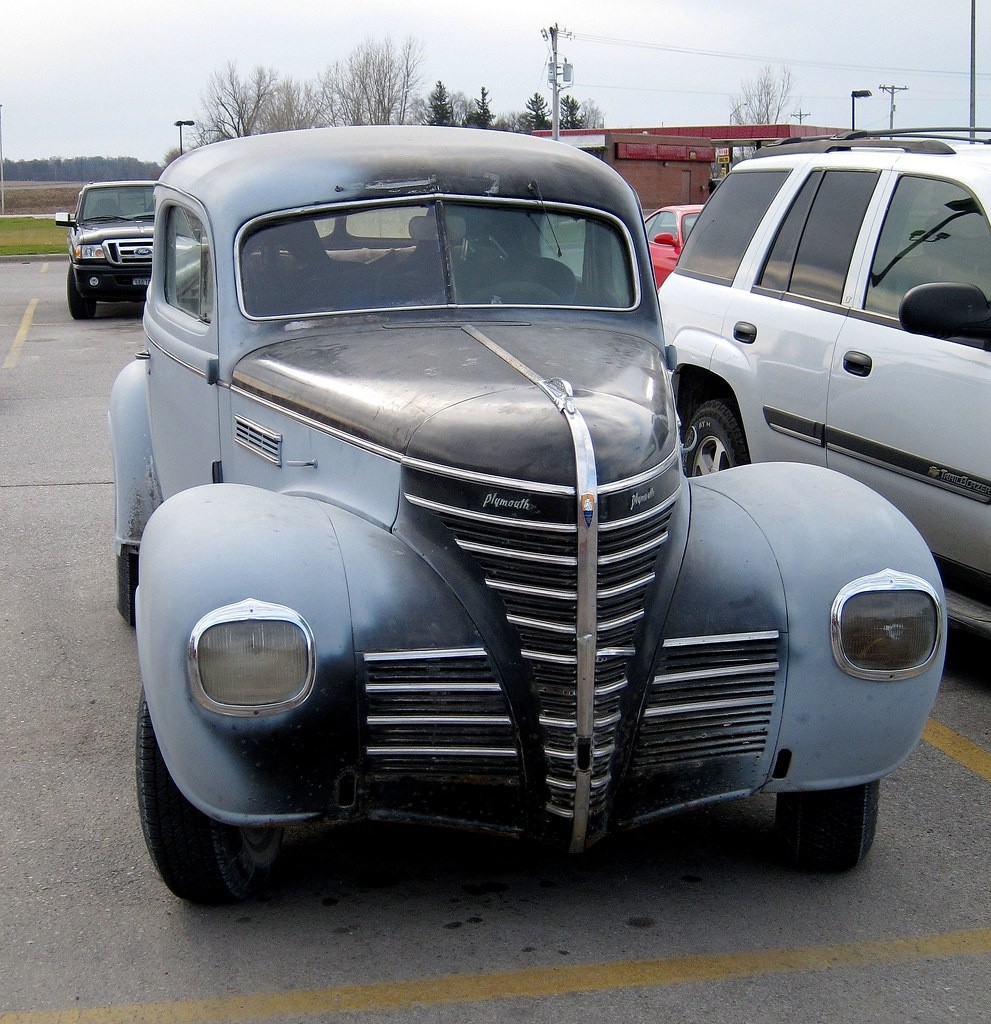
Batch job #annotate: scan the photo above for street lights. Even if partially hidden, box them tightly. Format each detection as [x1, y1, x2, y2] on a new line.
[173, 118, 195, 155]
[728, 102, 749, 126]
[849, 90, 874, 130]
[0, 104, 5, 215]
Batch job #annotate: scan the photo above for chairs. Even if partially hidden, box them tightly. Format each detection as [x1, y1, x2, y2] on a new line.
[90, 199, 125, 217]
[241, 216, 576, 311]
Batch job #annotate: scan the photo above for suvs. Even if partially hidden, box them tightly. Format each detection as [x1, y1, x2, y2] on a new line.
[54, 179, 200, 321]
[658, 126, 990, 644]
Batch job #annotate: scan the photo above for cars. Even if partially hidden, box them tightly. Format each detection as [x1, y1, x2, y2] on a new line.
[641, 204, 710, 289]
[104, 121, 955, 909]
